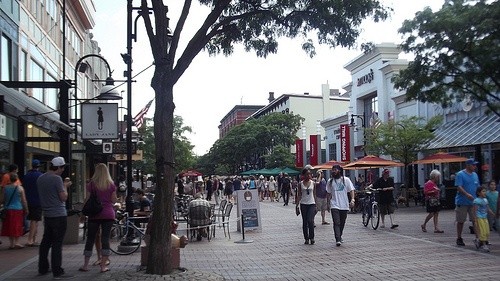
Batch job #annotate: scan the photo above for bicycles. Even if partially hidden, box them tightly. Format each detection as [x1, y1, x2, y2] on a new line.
[362, 188, 380, 230]
[109, 209, 144, 255]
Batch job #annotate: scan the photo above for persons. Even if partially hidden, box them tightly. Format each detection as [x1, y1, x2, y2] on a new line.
[37, 157, 74, 277]
[117, 175, 128, 203]
[366, 169, 399, 229]
[296, 165, 356, 246]
[0, 164, 30, 235]
[134, 190, 152, 235]
[79, 163, 117, 273]
[22, 160, 44, 245]
[177, 173, 297, 241]
[473, 186, 496, 253]
[2, 173, 29, 248]
[455, 159, 491, 246]
[420, 169, 444, 233]
[485, 180, 500, 235]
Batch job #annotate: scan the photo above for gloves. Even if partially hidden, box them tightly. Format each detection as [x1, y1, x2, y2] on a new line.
[350, 199, 355, 206]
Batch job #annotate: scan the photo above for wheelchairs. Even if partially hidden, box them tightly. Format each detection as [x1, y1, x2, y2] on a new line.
[189, 206, 213, 241]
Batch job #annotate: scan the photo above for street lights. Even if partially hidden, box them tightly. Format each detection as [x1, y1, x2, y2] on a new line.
[74, 54, 123, 142]
[118, 1, 174, 253]
[347, 111, 367, 185]
[121, 90, 126, 133]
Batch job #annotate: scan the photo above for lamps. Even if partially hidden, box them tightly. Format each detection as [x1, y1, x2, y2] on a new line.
[72, 54, 123, 144]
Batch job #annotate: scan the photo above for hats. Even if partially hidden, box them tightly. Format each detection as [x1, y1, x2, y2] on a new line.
[258, 175, 264, 179]
[382, 169, 391, 175]
[466, 159, 478, 165]
[51, 156, 70, 166]
[32, 159, 40, 166]
[11, 173, 17, 178]
[304, 165, 314, 170]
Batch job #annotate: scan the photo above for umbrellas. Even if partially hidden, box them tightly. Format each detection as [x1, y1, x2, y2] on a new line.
[251, 168, 275, 177]
[343, 154, 406, 179]
[281, 167, 300, 177]
[240, 170, 256, 175]
[179, 167, 203, 179]
[311, 159, 349, 174]
[271, 167, 282, 175]
[407, 152, 468, 184]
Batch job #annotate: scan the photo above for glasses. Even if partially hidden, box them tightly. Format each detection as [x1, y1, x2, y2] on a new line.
[306, 189, 309, 194]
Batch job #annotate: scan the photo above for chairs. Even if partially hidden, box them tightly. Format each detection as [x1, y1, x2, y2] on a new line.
[173, 193, 233, 242]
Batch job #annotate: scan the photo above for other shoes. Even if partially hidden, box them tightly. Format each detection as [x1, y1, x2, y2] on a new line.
[304, 241, 309, 244]
[434, 230, 444, 233]
[311, 240, 315, 245]
[473, 239, 492, 252]
[28, 242, 33, 245]
[421, 225, 427, 232]
[379, 224, 385, 228]
[336, 242, 341, 246]
[456, 238, 465, 246]
[341, 238, 344, 243]
[391, 224, 399, 229]
[322, 221, 330, 224]
[102, 268, 110, 272]
[15, 245, 25, 248]
[80, 266, 88, 271]
[33, 242, 41, 246]
[53, 268, 64, 277]
[469, 225, 475, 234]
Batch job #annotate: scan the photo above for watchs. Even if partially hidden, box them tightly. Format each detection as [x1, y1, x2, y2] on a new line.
[352, 199, 355, 201]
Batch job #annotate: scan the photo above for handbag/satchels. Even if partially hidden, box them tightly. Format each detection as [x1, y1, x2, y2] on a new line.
[0, 208, 7, 219]
[428, 194, 440, 206]
[82, 182, 103, 217]
